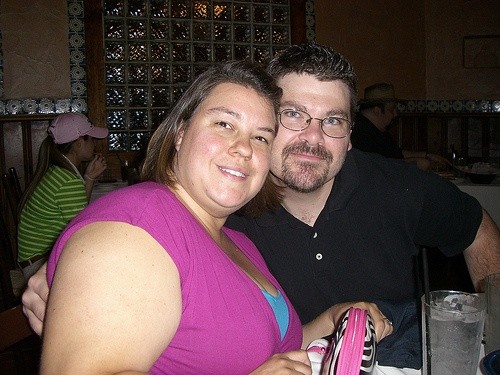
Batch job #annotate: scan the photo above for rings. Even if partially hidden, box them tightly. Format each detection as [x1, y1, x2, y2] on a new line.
[389, 322, 392, 324]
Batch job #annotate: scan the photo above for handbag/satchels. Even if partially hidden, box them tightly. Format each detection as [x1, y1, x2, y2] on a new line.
[321, 307, 376, 375]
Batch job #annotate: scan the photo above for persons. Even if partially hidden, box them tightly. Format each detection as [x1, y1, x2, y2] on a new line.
[351, 83, 404, 159]
[39, 57, 394, 375]
[18, 112, 109, 281]
[20, 41, 500, 375]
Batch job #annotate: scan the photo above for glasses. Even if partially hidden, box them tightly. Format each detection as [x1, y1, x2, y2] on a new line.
[276, 109, 355, 138]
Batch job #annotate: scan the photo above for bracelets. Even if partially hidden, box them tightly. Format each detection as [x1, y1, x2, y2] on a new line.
[84, 175, 95, 181]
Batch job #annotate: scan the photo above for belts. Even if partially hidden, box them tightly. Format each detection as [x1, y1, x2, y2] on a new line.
[19, 254, 46, 271]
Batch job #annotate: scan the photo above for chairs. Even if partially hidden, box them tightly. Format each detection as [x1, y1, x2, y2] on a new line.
[0, 166, 23, 268]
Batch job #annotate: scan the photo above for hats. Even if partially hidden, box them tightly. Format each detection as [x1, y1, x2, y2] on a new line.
[47, 112, 109, 145]
[358, 83, 404, 104]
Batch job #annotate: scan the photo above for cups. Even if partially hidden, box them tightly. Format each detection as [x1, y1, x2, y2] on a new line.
[421, 290, 487, 375]
[485, 272, 500, 355]
[127, 168, 138, 186]
[120, 159, 130, 181]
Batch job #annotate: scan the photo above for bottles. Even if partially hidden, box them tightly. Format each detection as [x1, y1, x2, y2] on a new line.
[449, 146, 466, 178]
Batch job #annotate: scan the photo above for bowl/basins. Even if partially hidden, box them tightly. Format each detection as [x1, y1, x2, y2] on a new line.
[483, 350, 500, 375]
[465, 172, 498, 184]
